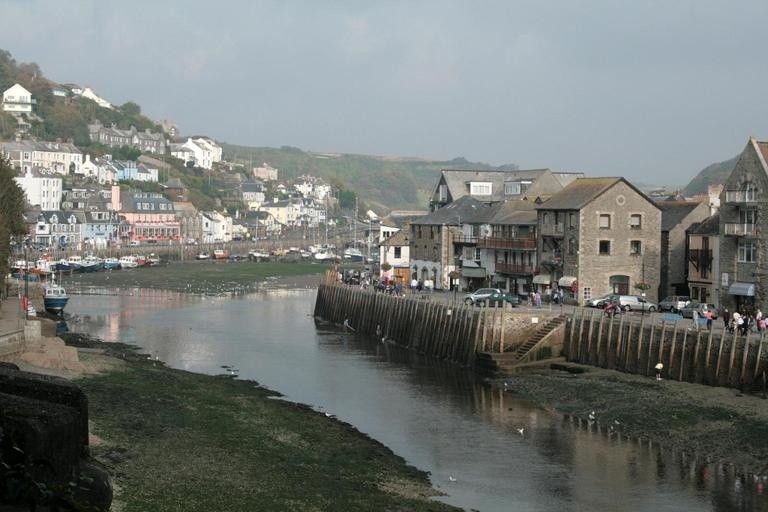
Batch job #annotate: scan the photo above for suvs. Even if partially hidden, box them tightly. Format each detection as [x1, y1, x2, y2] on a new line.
[463, 288, 501, 307]
[656, 296, 692, 313]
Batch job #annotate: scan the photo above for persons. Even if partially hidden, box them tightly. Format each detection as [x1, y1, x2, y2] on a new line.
[415, 278, 423, 291]
[688, 309, 699, 332]
[525, 285, 564, 307]
[410, 277, 417, 295]
[721, 307, 767, 337]
[705, 310, 713, 332]
[423, 279, 428, 292]
[653, 360, 665, 381]
[428, 278, 433, 292]
[340, 270, 403, 296]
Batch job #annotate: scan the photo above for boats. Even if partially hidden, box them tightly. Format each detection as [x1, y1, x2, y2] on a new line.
[9, 252, 165, 286]
[193, 241, 378, 265]
[40, 274, 71, 317]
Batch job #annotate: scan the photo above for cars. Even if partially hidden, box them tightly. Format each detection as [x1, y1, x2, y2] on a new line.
[371, 278, 402, 297]
[473, 292, 524, 308]
[10, 238, 158, 252]
[677, 302, 720, 320]
[231, 234, 246, 243]
[341, 268, 362, 285]
[585, 294, 618, 309]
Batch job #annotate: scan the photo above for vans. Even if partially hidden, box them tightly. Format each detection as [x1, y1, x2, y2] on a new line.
[616, 296, 658, 313]
